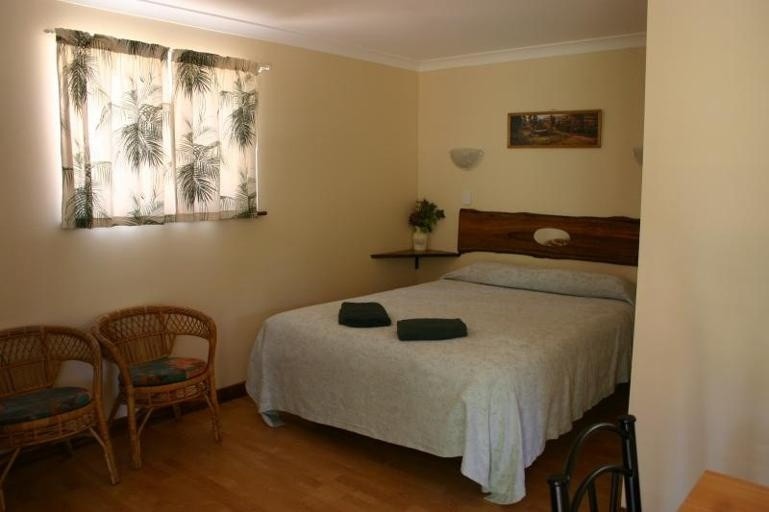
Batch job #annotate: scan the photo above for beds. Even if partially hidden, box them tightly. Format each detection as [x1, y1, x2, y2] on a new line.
[247, 209, 641, 504]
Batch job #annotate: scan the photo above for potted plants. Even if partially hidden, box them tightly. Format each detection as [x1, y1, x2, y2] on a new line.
[407, 198, 445, 251]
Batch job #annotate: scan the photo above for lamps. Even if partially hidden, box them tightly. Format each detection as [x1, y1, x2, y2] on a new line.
[633, 145, 644, 165]
[450, 148, 484, 169]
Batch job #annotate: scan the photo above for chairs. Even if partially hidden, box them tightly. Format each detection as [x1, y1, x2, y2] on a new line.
[0, 325, 120, 512]
[91, 304, 223, 470]
[546, 414, 640, 512]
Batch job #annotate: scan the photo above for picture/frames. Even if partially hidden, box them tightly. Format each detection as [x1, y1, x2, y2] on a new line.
[507, 109, 602, 149]
